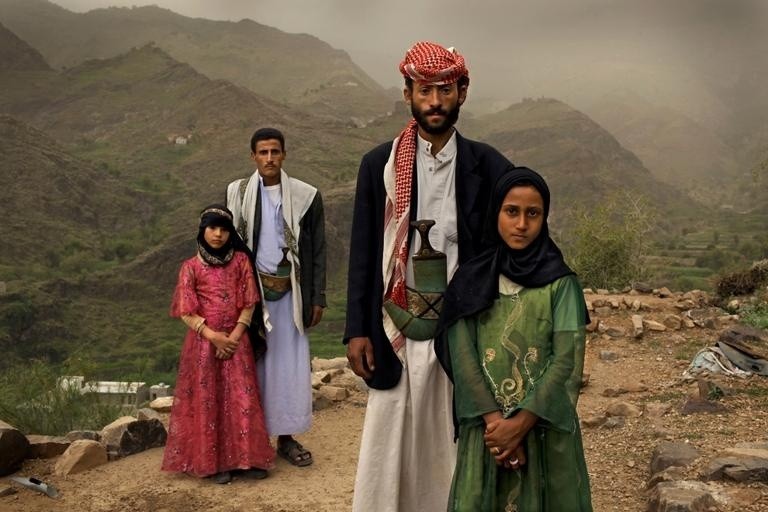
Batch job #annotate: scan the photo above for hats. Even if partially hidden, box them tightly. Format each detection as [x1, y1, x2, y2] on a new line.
[399, 42, 467, 85]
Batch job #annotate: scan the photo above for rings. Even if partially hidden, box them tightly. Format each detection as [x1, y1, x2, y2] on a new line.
[510, 458, 518, 465]
[495, 447, 500, 453]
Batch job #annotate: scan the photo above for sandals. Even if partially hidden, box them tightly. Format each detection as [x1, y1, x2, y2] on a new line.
[277, 436, 313, 466]
[215, 468, 268, 484]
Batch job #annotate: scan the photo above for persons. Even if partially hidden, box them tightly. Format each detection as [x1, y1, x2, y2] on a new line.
[431, 165, 595, 512]
[225, 127, 328, 467]
[160, 205, 277, 485]
[341, 43, 514, 510]
